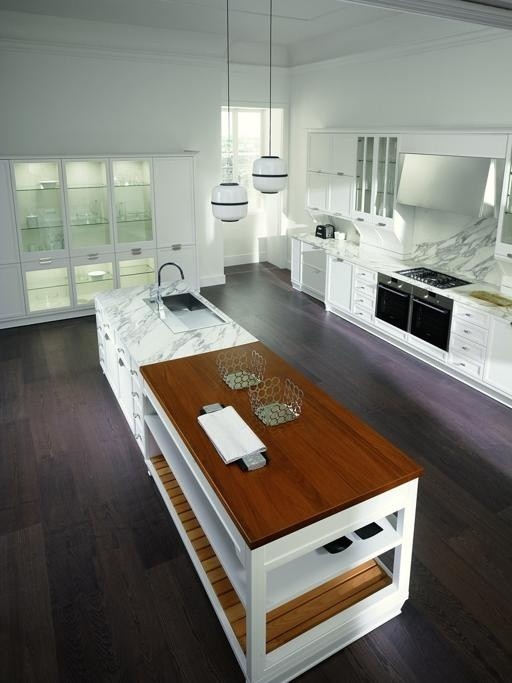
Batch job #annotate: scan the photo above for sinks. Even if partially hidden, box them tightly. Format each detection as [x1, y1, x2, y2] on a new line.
[144, 292, 207, 312]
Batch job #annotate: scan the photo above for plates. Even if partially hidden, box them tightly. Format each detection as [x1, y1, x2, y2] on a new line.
[323, 535, 352, 554]
[355, 521, 383, 540]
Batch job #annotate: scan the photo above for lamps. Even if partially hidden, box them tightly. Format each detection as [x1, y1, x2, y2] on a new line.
[210, 0, 249, 223]
[251, 0, 289, 195]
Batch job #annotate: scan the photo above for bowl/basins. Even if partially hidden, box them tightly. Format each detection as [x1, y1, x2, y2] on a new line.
[88, 270, 106, 281]
[40, 180, 59, 189]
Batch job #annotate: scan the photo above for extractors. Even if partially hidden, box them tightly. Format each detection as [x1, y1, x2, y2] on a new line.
[396, 152, 499, 218]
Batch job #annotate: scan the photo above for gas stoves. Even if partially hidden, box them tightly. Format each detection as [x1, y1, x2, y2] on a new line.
[394, 266, 472, 290]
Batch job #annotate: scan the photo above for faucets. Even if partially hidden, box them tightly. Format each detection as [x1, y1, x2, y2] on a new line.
[157, 261, 185, 304]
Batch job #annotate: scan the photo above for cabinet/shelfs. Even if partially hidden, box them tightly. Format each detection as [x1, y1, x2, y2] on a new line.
[95, 300, 145, 460]
[494, 131, 512, 288]
[143, 382, 419, 683]
[353, 132, 422, 254]
[290, 238, 512, 407]
[305, 131, 357, 221]
[0, 150, 201, 330]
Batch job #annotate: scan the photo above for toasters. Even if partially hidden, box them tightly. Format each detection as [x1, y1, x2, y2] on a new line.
[314, 224, 335, 240]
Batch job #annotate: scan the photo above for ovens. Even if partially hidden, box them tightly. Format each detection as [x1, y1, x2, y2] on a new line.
[405, 283, 456, 351]
[375, 272, 411, 332]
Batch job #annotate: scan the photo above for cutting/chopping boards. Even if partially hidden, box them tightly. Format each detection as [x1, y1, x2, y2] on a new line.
[470, 290, 512, 308]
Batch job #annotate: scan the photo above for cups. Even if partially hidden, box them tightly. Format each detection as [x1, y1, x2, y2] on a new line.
[339, 232, 346, 241]
[114, 174, 144, 186]
[334, 231, 340, 239]
[25, 216, 39, 228]
